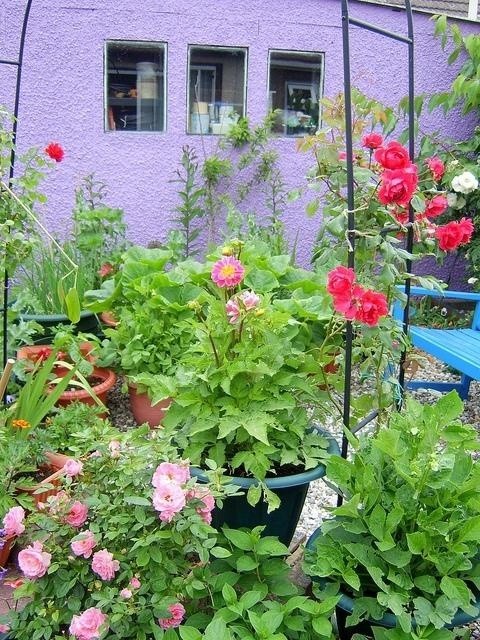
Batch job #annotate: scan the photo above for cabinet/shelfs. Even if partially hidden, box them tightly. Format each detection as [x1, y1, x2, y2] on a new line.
[108, 67, 163, 131]
